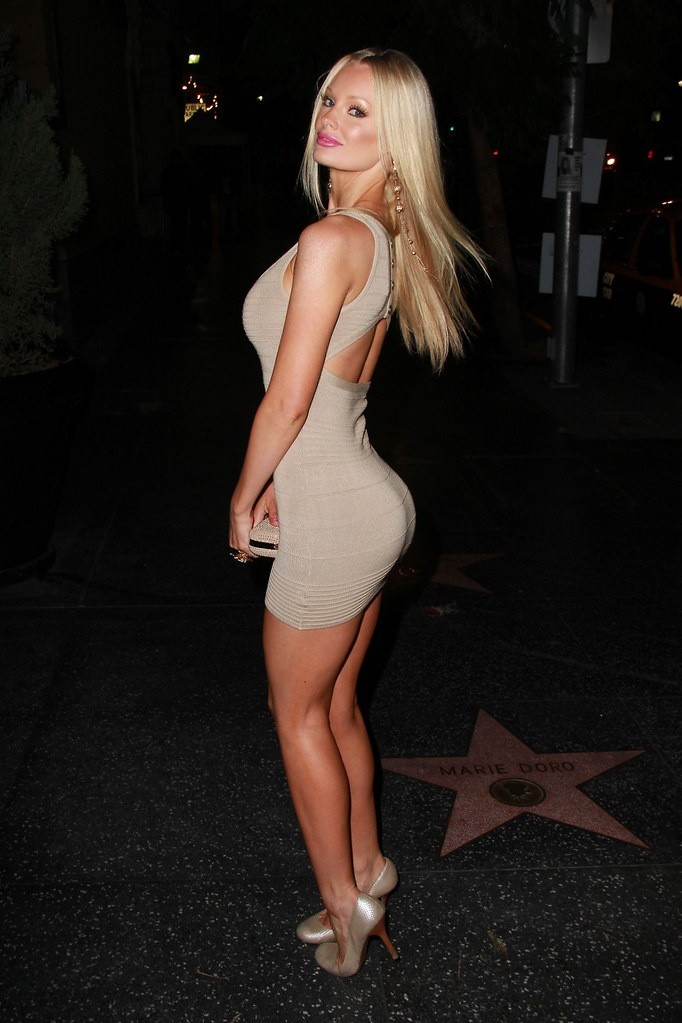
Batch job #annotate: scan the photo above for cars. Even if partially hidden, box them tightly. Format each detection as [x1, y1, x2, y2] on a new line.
[580, 194, 682, 358]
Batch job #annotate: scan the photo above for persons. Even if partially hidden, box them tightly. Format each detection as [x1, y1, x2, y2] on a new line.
[228, 49, 488, 978]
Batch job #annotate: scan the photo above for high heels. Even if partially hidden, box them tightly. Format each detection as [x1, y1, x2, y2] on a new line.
[315, 891, 399, 978]
[297, 856, 398, 944]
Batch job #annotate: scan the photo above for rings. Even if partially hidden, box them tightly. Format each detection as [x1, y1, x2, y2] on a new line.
[230, 551, 251, 563]
